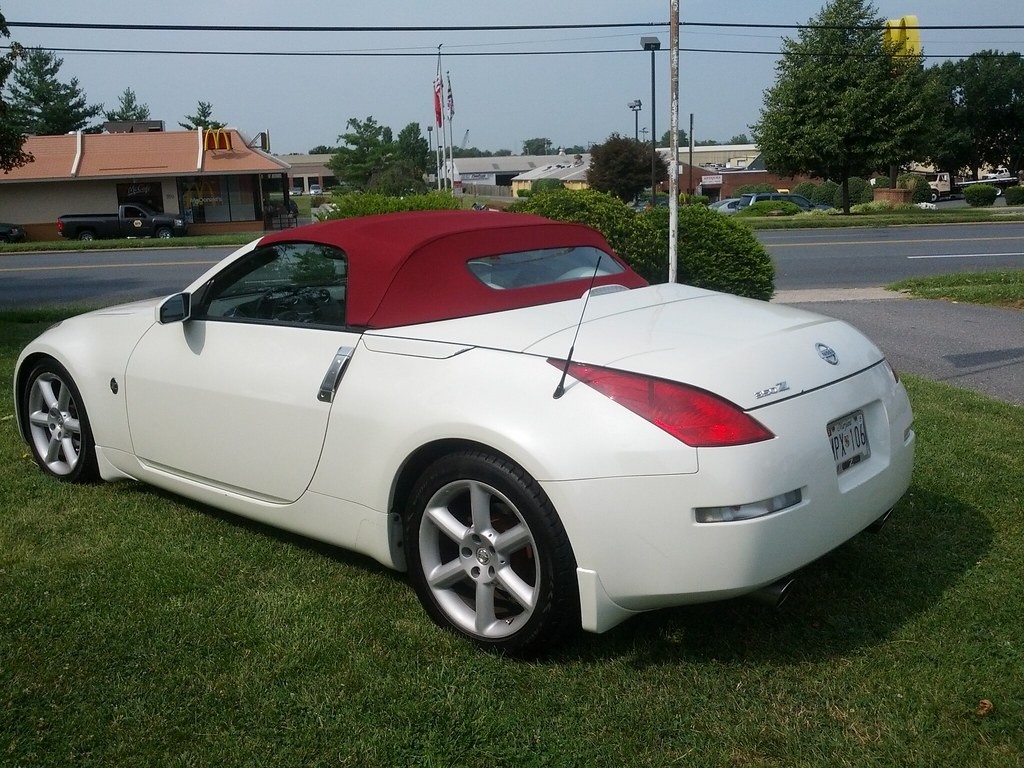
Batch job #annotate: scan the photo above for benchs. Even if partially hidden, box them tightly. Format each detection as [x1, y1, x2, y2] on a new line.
[487, 266, 612, 289]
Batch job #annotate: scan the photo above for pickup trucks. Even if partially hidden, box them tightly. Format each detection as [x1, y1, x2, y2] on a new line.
[57, 202, 188, 241]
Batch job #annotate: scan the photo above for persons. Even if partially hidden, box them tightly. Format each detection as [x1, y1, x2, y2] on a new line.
[679, 189, 686, 203]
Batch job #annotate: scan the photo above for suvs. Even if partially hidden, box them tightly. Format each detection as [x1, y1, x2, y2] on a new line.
[736, 192, 831, 214]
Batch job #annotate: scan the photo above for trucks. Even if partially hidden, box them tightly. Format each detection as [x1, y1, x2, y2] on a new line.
[924, 172, 1019, 202]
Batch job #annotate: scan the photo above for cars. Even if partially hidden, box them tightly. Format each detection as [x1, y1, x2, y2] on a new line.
[982, 167, 1010, 180]
[288, 187, 303, 196]
[309, 184, 323, 196]
[706, 198, 740, 215]
[13, 209, 915, 663]
[0, 222, 29, 242]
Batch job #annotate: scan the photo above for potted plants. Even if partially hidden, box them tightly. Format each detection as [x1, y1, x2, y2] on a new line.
[310, 195, 325, 224]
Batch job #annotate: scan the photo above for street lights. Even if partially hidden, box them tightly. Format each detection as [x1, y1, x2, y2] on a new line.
[626, 100, 642, 146]
[427, 126, 433, 151]
[639, 36, 661, 208]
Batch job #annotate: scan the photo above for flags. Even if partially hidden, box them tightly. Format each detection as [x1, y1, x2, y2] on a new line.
[434, 75, 445, 128]
[447, 75, 454, 119]
